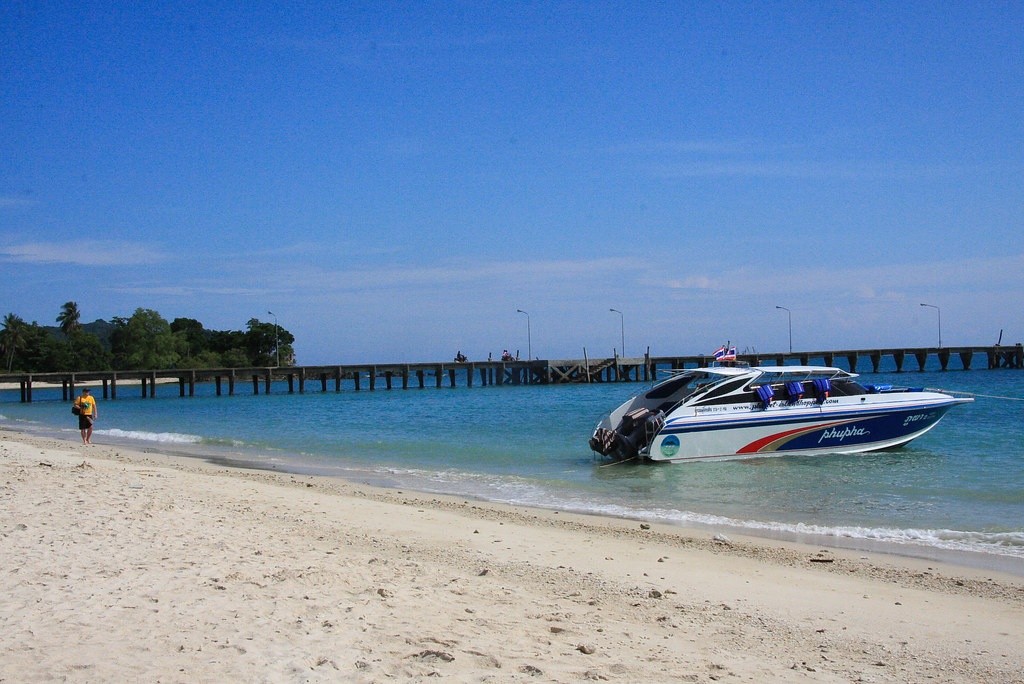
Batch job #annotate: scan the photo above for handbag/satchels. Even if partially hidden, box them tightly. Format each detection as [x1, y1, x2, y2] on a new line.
[72, 407, 80, 415]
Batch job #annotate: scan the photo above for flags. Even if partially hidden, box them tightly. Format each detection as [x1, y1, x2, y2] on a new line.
[712, 347, 725, 358]
[727, 346, 736, 355]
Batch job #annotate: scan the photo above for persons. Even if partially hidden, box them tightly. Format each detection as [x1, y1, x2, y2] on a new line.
[502, 350, 510, 361]
[457, 351, 462, 363]
[74, 387, 98, 446]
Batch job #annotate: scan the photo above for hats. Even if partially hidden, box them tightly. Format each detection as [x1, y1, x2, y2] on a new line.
[83, 388, 91, 391]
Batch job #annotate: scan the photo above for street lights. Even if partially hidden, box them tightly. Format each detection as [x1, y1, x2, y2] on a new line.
[920, 303, 942, 349]
[517, 309, 531, 360]
[610, 308, 625, 358]
[776, 306, 792, 354]
[267, 310, 279, 367]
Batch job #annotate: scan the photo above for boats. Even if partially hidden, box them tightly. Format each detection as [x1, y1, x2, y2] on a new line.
[588, 362, 976, 464]
